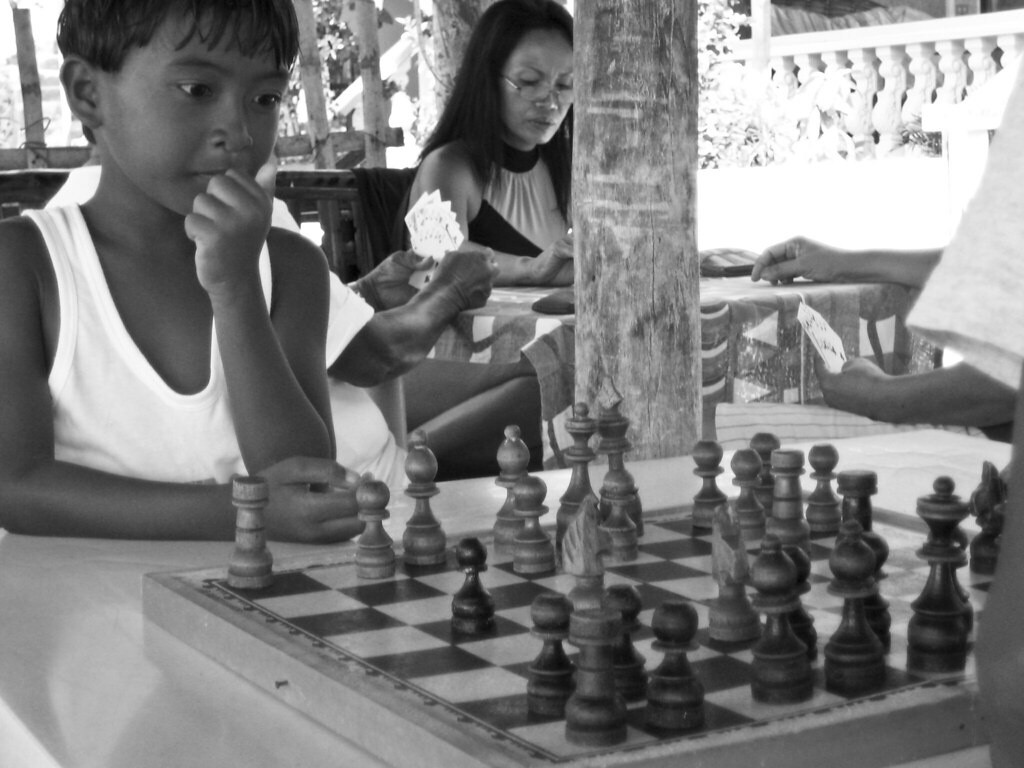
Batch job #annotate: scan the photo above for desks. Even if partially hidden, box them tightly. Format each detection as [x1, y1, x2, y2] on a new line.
[0, 422, 1023, 768]
[401, 272, 941, 474]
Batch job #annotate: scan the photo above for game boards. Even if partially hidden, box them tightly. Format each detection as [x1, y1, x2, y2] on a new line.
[142, 487, 1004, 767]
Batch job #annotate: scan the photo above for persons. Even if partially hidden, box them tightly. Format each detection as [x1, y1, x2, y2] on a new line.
[0, 0, 375, 543]
[43, 146, 542, 490]
[905, 57, 1024, 768]
[392, 0, 574, 286]
[751, 235, 1017, 426]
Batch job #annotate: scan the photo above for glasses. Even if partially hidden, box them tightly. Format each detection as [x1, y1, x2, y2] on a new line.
[498, 74, 575, 105]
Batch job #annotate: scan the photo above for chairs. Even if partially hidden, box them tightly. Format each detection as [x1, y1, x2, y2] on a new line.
[273, 171, 376, 287]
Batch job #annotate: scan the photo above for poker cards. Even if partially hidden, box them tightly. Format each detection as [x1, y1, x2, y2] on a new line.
[797, 301, 847, 374]
[403, 186, 467, 291]
